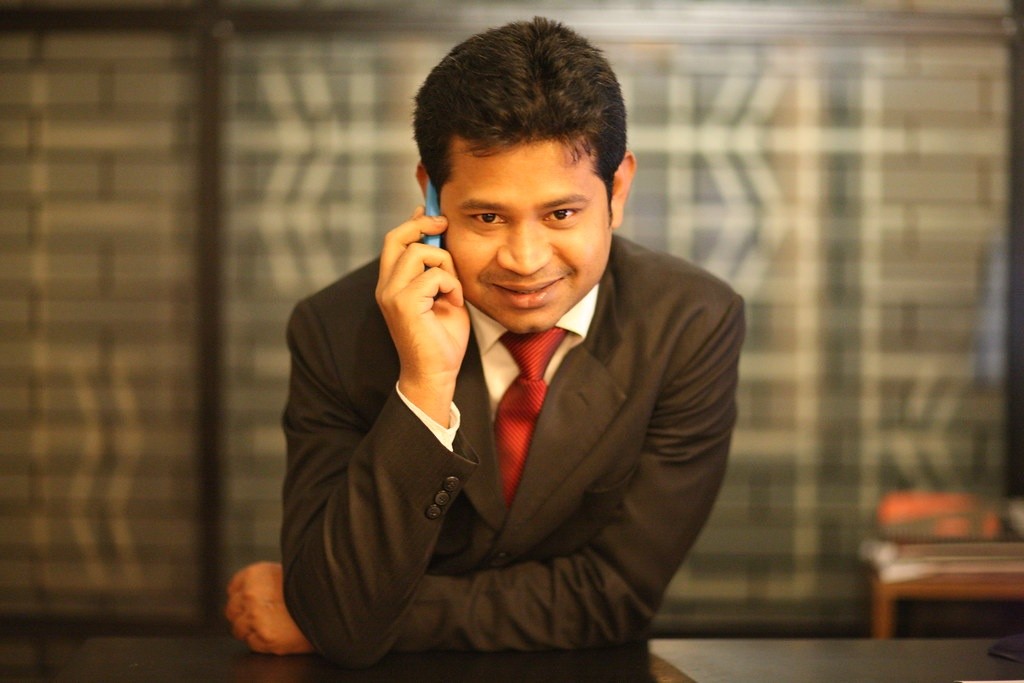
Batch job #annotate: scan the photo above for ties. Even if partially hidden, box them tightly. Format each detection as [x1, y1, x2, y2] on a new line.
[495, 327, 566, 514]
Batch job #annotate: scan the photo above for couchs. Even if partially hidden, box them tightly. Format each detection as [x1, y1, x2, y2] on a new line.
[864, 491, 1024, 637]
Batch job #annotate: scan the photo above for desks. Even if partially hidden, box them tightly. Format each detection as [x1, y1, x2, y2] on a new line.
[647, 635, 1024, 683]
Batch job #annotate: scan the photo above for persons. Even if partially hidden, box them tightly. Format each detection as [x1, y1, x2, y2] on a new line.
[226, 15, 746, 671]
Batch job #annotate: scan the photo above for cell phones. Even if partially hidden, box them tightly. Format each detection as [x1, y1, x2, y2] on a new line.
[423, 182, 442, 270]
